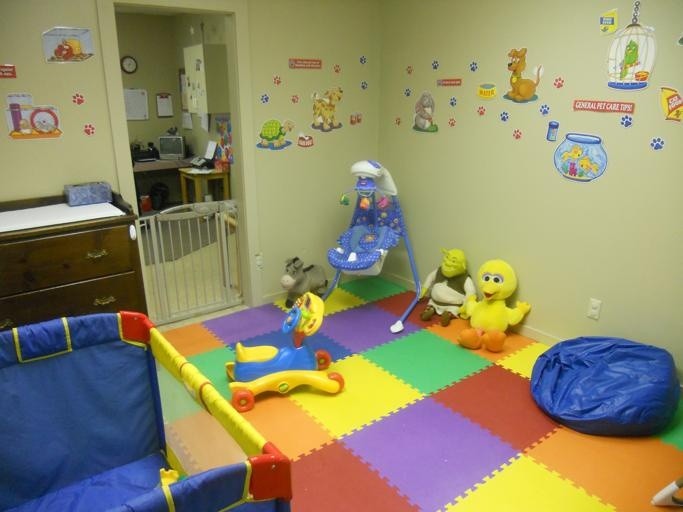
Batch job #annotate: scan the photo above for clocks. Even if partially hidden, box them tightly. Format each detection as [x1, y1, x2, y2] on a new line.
[121, 56, 137, 73]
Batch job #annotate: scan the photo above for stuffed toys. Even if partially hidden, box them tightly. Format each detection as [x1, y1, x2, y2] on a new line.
[281, 256, 328, 308]
[455, 259, 532, 352]
[417, 246, 474, 326]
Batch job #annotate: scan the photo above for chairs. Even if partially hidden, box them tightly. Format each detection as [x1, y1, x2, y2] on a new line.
[316, 161, 422, 333]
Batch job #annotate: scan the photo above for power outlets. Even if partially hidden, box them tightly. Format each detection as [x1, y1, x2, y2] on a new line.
[588, 298, 602, 320]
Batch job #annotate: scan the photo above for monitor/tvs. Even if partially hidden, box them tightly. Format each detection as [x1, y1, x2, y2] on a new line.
[158, 136, 189, 161]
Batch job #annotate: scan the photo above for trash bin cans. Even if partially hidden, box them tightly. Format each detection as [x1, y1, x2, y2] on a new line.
[151, 183, 168, 209]
[140, 192, 152, 211]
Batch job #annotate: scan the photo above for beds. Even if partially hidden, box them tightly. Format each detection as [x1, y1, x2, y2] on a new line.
[0, 311, 292, 512]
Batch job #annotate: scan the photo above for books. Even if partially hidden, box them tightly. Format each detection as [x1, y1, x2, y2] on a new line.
[204, 140, 217, 160]
[155, 93, 173, 117]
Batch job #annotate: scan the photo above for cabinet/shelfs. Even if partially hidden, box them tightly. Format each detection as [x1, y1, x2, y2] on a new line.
[183, 44, 229, 115]
[1, 225, 149, 331]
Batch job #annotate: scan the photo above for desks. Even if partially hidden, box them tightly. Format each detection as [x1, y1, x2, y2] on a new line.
[133, 158, 230, 222]
